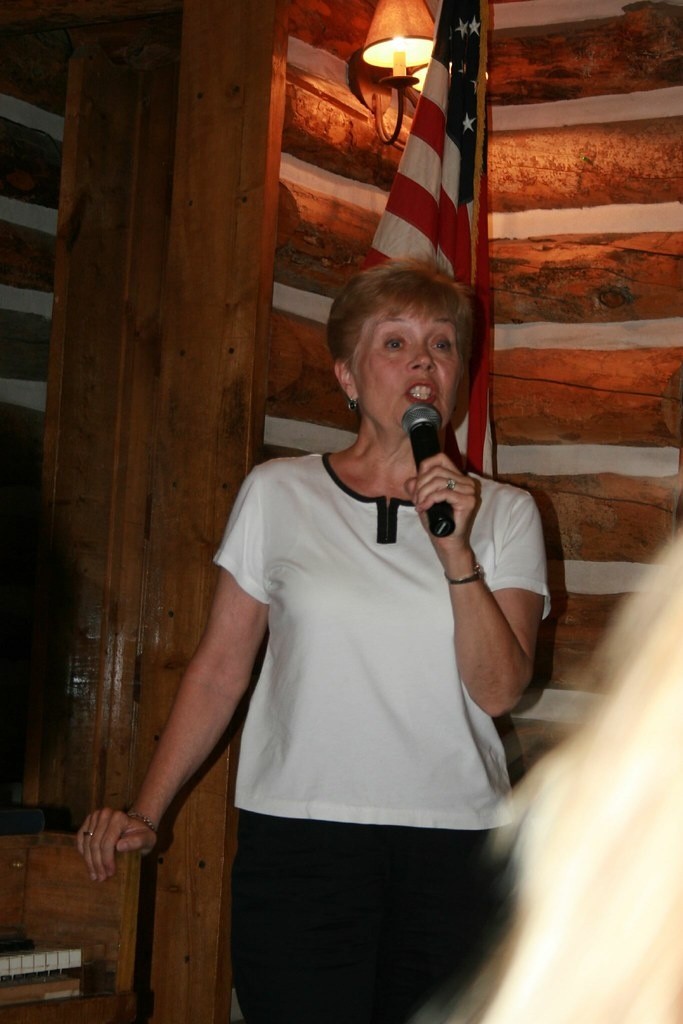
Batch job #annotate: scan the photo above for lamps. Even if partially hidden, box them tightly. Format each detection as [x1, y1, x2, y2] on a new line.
[348, 0, 437, 145]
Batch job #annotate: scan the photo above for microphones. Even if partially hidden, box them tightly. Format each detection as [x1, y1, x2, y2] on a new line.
[402, 402, 456, 537]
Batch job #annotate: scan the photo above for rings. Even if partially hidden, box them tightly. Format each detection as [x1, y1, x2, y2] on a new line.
[84, 832, 93, 835]
[447, 479, 455, 489]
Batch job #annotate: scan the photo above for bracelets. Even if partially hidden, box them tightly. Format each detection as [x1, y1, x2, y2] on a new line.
[444, 563, 482, 584]
[128, 813, 156, 832]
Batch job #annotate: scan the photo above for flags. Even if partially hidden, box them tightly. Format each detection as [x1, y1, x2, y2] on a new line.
[363, 0, 493, 477]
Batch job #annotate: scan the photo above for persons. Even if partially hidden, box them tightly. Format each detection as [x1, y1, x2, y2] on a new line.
[77, 263, 551, 1024]
